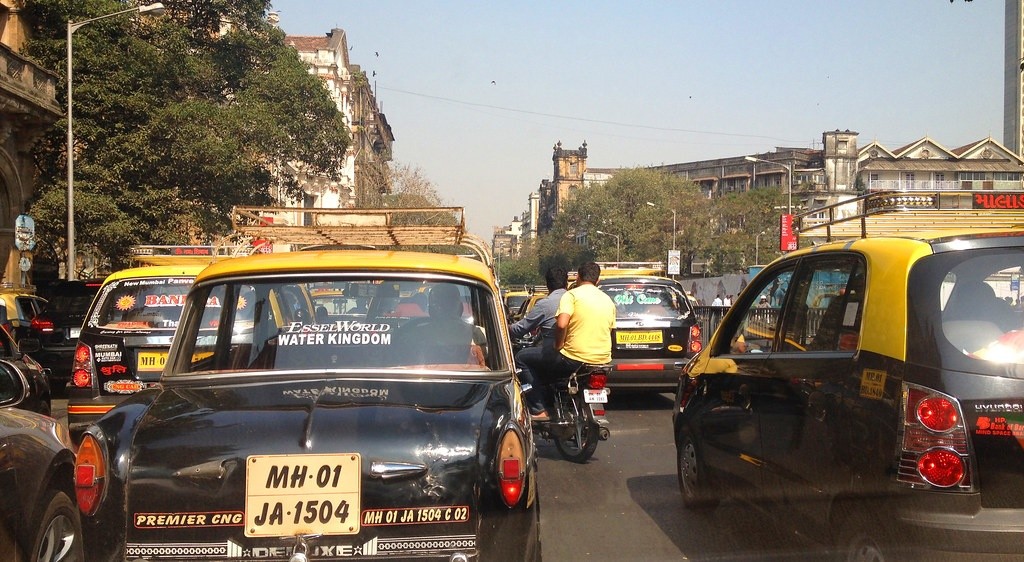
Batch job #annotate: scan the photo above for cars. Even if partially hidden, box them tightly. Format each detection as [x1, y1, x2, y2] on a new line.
[0, 201, 709, 562]
[668, 226, 1020, 562]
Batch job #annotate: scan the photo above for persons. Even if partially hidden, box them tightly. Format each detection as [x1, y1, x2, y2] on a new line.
[712, 294, 733, 306]
[757, 295, 772, 323]
[386, 284, 486, 366]
[507, 261, 616, 421]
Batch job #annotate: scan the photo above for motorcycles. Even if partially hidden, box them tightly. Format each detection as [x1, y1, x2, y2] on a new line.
[507, 315, 611, 463]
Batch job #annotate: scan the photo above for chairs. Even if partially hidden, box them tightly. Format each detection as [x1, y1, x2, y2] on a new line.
[808, 295, 863, 350]
[949, 299, 1017, 330]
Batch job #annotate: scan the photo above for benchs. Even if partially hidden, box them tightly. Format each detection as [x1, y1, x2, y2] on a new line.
[942, 320, 1004, 354]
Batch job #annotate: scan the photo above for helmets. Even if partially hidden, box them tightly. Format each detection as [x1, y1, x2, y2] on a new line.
[761, 295, 766, 298]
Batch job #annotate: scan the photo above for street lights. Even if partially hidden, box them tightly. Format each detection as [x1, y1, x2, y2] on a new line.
[596, 230, 620, 267]
[745, 156, 790, 227]
[65, 1, 167, 282]
[648, 202, 677, 281]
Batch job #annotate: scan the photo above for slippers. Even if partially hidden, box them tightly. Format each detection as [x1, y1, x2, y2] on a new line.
[531, 410, 551, 421]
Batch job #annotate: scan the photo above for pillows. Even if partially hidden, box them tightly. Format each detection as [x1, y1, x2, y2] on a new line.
[963, 326, 1024, 365]
